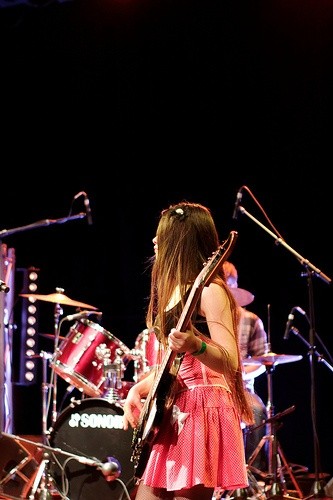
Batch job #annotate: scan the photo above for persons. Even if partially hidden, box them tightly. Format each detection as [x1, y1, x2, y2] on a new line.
[123, 202, 256, 500]
[222, 262, 270, 482]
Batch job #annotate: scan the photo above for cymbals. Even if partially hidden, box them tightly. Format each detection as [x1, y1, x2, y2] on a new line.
[18, 291, 99, 311]
[240, 353, 304, 368]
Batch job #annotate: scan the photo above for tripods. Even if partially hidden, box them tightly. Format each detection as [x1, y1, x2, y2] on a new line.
[245, 366, 305, 499]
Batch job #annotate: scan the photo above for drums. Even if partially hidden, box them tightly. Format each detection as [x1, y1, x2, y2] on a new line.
[47, 398, 148, 500]
[132, 327, 167, 384]
[48, 318, 132, 398]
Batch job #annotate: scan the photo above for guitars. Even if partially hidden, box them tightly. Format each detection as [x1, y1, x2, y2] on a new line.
[130, 230, 238, 479]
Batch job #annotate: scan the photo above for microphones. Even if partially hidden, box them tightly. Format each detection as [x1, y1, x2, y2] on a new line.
[232, 191, 243, 219]
[98, 460, 120, 476]
[83, 195, 92, 225]
[284, 308, 296, 339]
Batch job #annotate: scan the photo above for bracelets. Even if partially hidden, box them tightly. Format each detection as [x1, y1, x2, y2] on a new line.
[190, 341, 206, 356]
